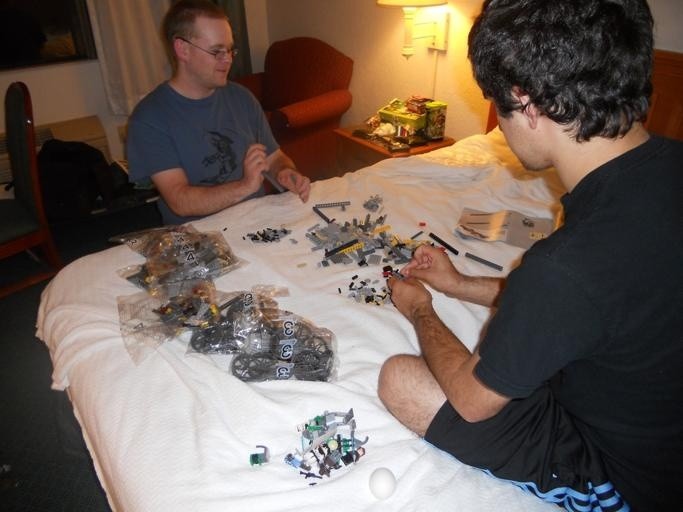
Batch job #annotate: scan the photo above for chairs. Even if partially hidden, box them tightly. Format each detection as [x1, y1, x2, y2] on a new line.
[236, 37, 354, 194]
[2, 81, 55, 294]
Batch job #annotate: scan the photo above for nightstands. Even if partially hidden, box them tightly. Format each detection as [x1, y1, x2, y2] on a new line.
[334, 126, 456, 178]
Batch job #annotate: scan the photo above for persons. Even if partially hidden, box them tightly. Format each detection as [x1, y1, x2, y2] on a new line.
[372, 0, 683, 510]
[124, 0, 312, 226]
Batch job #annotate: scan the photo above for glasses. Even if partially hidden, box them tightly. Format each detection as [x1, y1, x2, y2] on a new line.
[173, 35, 240, 62]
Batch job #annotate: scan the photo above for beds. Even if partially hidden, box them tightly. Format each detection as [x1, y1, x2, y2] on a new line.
[48, 42, 682, 507]
